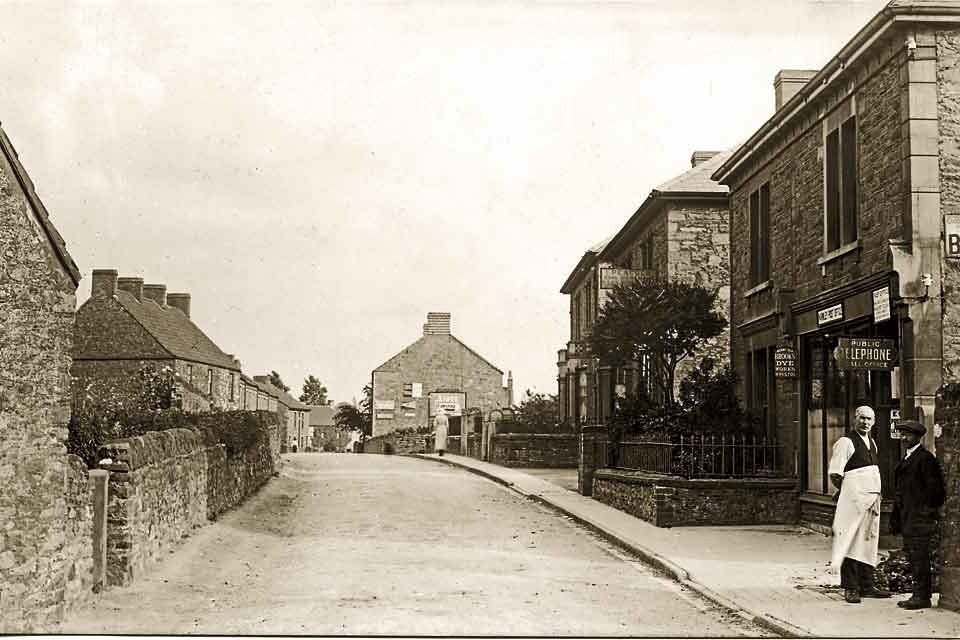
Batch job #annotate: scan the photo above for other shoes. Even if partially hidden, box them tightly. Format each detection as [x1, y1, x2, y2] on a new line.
[864, 587, 891, 597]
[439, 450, 443, 456]
[897, 599, 931, 609]
[845, 589, 860, 603]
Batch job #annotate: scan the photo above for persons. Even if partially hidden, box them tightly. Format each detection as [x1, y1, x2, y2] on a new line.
[433, 407, 450, 458]
[828, 405, 892, 603]
[893, 421, 946, 611]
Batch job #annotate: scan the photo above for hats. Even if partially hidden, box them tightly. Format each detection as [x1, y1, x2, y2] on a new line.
[896, 420, 926, 437]
